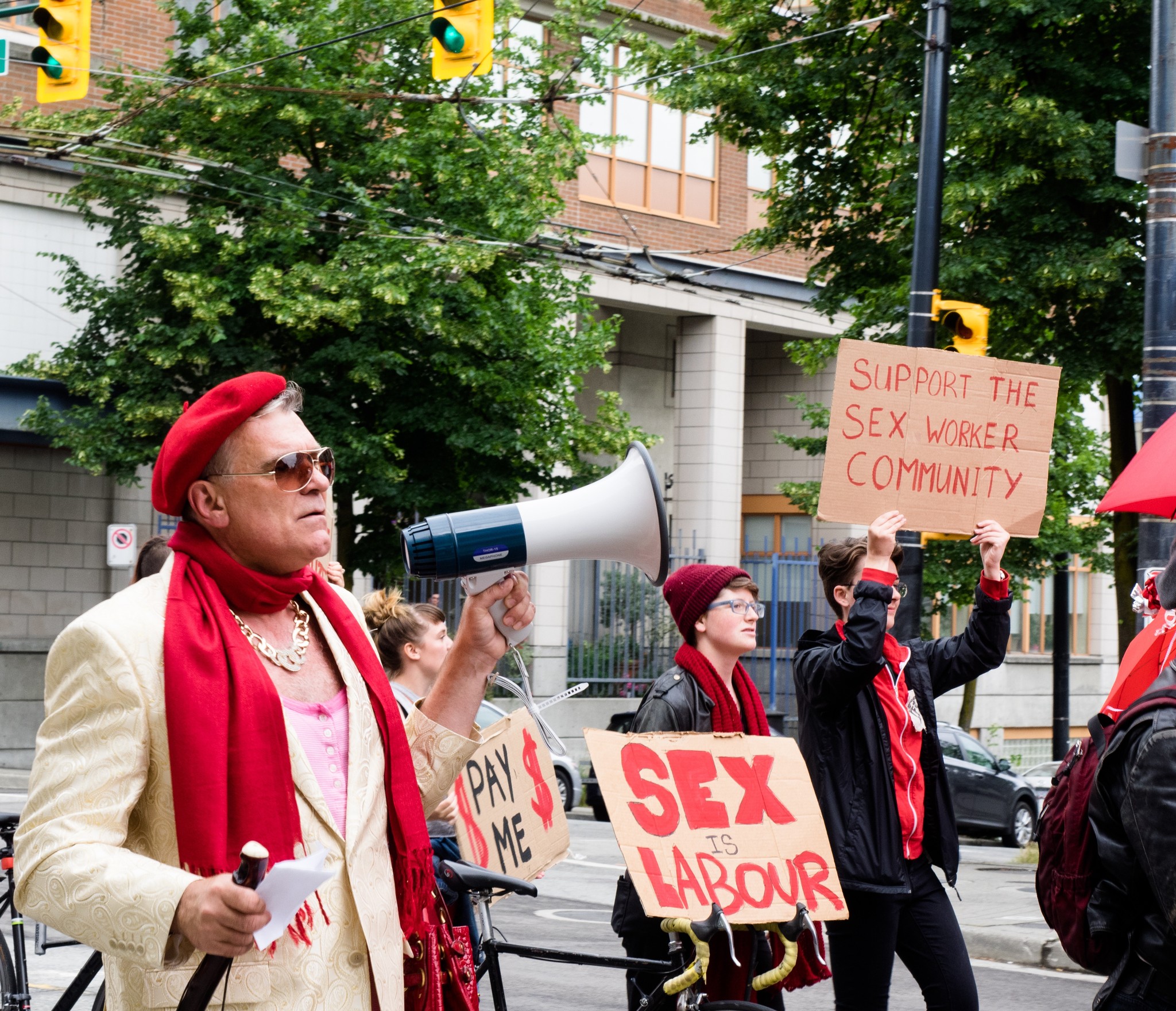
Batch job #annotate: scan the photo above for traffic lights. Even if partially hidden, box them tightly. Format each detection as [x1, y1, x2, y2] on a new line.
[430, 14, 476, 56]
[941, 311, 980, 356]
[30, 0, 76, 83]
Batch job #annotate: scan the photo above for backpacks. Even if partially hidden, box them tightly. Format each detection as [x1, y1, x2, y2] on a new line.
[1035, 684, 1176, 976]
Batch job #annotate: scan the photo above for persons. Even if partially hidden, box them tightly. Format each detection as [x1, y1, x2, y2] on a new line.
[10, 371, 537, 1011]
[130, 534, 174, 589]
[427, 591, 441, 606]
[793, 508, 1015, 1011]
[358, 588, 496, 981]
[1089, 554, 1176, 1011]
[612, 563, 832, 1011]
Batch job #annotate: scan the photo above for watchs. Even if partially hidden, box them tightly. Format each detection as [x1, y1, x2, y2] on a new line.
[1000, 570, 1006, 580]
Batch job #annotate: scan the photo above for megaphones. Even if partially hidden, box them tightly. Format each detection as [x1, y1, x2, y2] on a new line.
[398, 439, 672, 648]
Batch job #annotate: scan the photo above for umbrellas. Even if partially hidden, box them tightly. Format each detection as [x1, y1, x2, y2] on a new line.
[1093, 410, 1176, 516]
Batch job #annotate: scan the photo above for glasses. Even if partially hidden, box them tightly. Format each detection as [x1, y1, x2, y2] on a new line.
[202, 446, 337, 493]
[840, 583, 908, 597]
[703, 598, 767, 618]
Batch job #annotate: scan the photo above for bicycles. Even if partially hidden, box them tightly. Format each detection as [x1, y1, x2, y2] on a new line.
[0, 808, 107, 1011]
[437, 857, 828, 1011]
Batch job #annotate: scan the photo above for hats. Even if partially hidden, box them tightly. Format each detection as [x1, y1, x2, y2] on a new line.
[663, 565, 752, 643]
[147, 370, 290, 514]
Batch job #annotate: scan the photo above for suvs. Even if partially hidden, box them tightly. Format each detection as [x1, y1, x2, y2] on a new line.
[476, 702, 1063, 846]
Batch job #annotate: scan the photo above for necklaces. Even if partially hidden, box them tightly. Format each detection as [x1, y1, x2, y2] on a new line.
[218, 597, 311, 672]
